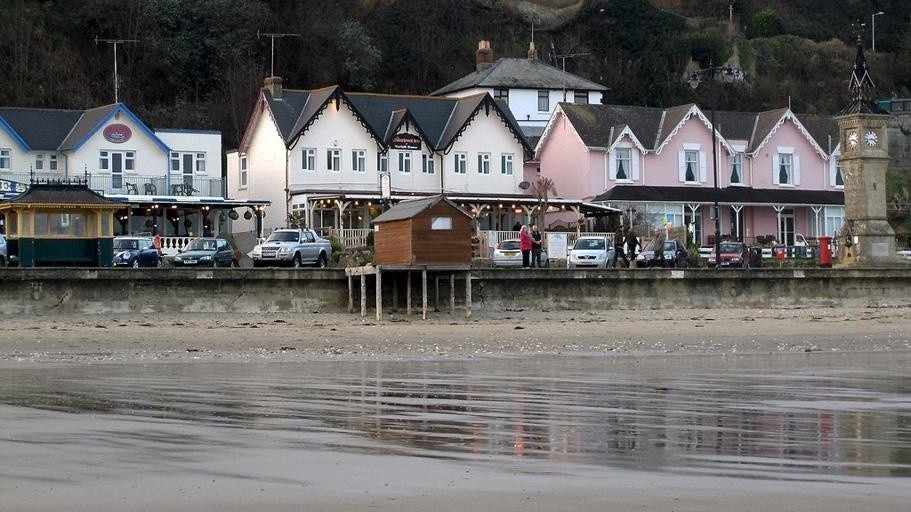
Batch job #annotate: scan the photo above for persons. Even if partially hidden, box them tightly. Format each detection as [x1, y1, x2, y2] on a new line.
[519, 224, 532, 269]
[651, 227, 671, 267]
[612, 225, 631, 269]
[513, 221, 522, 232]
[530, 225, 544, 269]
[621, 225, 642, 261]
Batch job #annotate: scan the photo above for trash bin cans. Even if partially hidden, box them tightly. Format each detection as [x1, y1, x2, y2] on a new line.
[749, 246, 762, 268]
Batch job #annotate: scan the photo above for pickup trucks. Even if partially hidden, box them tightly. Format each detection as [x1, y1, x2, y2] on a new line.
[253, 226, 334, 271]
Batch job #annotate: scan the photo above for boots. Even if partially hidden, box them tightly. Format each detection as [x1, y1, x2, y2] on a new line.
[628, 260, 637, 269]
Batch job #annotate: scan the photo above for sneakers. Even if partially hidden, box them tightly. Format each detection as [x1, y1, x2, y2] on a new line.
[530, 266, 535, 268]
[611, 265, 617, 269]
[523, 266, 531, 268]
[538, 266, 544, 268]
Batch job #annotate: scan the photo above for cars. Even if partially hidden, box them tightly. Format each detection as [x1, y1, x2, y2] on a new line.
[0, 236, 9, 268]
[172, 236, 236, 271]
[491, 238, 552, 267]
[567, 232, 756, 269]
[112, 236, 164, 270]
